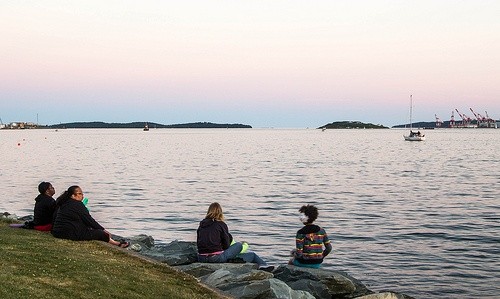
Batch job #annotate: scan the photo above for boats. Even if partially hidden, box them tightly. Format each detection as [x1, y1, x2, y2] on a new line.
[144, 122, 149, 131]
[423, 127, 434, 129]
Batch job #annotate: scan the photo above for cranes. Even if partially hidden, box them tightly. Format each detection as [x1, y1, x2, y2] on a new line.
[448, 108, 500, 128]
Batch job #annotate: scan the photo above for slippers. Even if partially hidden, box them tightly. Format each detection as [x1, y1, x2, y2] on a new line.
[118, 241, 130, 248]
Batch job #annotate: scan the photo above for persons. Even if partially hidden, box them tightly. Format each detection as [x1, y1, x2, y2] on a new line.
[34, 181, 56, 231]
[288, 204, 332, 269]
[52, 186, 129, 248]
[196, 202, 275, 271]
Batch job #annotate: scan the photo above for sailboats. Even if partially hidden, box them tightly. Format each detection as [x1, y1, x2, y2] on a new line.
[403, 94, 426, 140]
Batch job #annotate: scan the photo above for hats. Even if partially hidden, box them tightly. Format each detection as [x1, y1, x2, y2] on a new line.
[129, 243, 143, 253]
